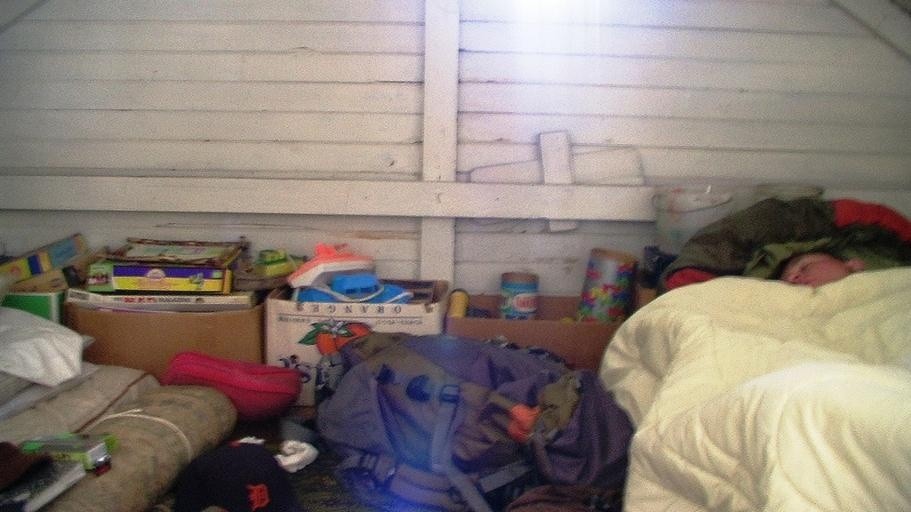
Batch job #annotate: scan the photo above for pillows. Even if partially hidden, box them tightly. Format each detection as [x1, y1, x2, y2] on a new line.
[0, 308, 97, 384]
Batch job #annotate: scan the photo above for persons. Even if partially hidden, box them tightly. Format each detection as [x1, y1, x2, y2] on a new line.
[772, 245, 867, 293]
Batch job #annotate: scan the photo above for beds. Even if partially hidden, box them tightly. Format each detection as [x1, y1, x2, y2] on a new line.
[602, 249, 910, 511]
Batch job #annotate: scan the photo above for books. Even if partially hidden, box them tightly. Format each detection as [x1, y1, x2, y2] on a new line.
[0, 457, 87, 511]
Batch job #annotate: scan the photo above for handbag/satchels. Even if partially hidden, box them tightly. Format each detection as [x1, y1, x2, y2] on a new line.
[315, 333, 633, 512]
[155, 353, 301, 422]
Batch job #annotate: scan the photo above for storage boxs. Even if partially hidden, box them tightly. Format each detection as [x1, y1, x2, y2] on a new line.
[262, 273, 451, 417]
[0, 232, 94, 325]
[61, 279, 268, 375]
[444, 292, 664, 370]
